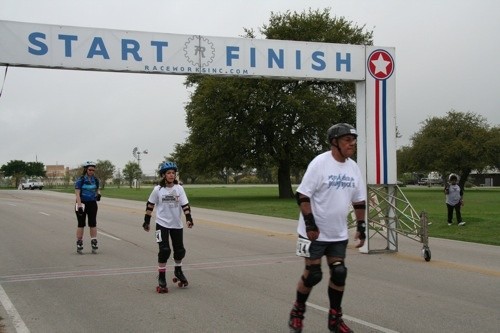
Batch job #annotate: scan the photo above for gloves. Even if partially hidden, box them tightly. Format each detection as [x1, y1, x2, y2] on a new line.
[95, 194, 101, 201]
[78, 208, 83, 215]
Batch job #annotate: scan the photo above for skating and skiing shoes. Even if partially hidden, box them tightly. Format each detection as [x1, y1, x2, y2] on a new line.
[76, 240, 83, 254]
[289, 304, 306, 332]
[91, 239, 98, 254]
[173, 270, 188, 287]
[156, 272, 169, 293]
[328, 308, 353, 333]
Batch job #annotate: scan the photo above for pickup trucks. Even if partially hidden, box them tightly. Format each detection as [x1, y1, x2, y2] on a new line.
[21, 179, 45, 191]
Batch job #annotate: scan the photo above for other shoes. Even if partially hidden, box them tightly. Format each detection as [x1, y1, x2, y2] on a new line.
[447, 223, 451, 226]
[458, 222, 466, 226]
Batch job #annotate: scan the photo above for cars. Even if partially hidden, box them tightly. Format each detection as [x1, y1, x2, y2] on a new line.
[418, 177, 438, 186]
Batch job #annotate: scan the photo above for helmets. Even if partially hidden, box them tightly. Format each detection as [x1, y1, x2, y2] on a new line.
[159, 161, 177, 174]
[326, 123, 358, 142]
[449, 173, 458, 181]
[83, 161, 96, 168]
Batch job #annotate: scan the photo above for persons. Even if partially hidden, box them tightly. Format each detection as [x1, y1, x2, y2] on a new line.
[444, 173, 466, 227]
[75, 162, 101, 254]
[142, 162, 194, 292]
[288, 123, 367, 333]
